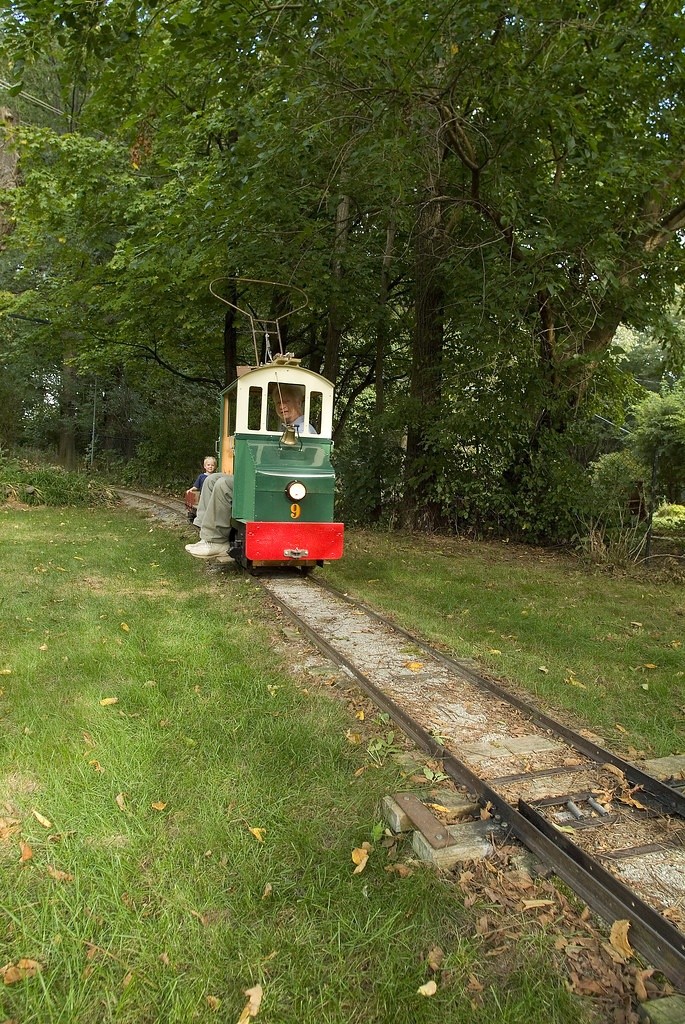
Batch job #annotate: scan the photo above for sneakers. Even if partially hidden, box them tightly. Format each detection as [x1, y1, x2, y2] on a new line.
[184, 539, 231, 558]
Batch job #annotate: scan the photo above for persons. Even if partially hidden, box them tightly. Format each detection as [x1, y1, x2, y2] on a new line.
[184, 385, 317, 558]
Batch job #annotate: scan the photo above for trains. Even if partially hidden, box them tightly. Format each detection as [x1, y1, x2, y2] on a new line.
[184, 277, 344, 581]
